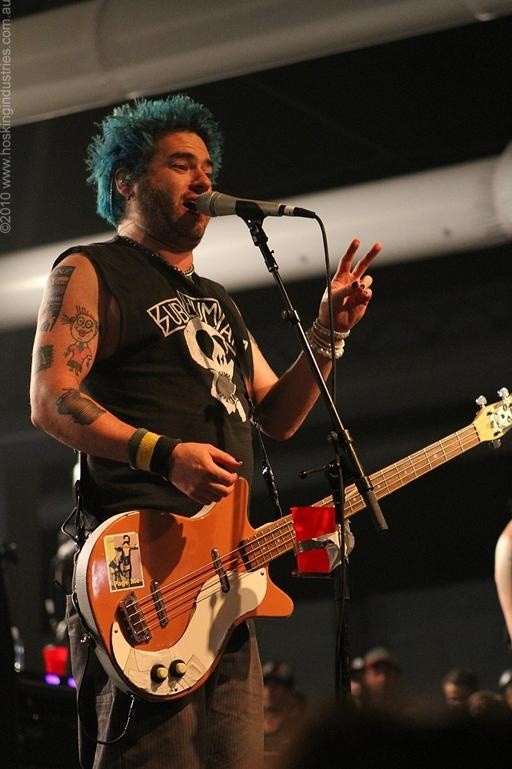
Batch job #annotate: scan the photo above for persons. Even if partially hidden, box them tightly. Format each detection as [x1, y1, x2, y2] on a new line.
[25, 94, 382, 769]
[442, 662, 512, 718]
[350, 648, 399, 705]
[261, 659, 303, 733]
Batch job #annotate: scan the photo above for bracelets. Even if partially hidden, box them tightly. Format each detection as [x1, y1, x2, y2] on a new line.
[126, 427, 183, 483]
[304, 317, 353, 362]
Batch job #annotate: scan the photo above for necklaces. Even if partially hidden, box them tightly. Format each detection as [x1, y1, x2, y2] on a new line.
[119, 235, 196, 278]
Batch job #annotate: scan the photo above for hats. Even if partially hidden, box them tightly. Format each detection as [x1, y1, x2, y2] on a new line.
[365, 648, 400, 673]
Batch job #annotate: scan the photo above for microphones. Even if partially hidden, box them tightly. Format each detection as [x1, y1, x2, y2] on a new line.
[198, 192, 315, 217]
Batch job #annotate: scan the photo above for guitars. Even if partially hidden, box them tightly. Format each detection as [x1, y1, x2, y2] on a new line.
[71, 386, 512, 703]
